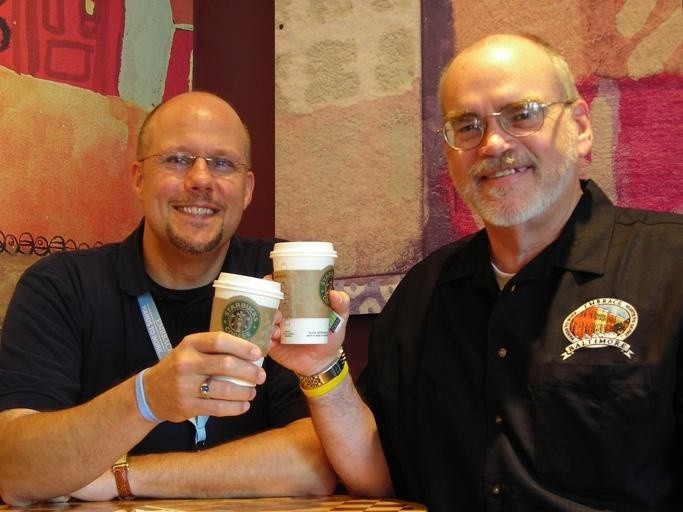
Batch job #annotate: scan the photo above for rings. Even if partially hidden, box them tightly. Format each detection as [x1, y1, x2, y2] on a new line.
[200, 377, 212, 398]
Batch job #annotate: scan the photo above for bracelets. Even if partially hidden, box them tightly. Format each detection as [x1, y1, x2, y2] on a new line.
[301, 361, 349, 398]
[135, 367, 164, 423]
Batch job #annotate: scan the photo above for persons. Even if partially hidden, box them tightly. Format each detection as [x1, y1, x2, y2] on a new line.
[263, 32, 681, 511]
[0, 90, 337, 507]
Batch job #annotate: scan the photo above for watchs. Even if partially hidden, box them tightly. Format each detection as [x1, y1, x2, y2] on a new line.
[295, 350, 346, 390]
[112, 452, 135, 502]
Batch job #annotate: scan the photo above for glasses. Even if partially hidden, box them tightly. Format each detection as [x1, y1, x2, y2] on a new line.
[437, 99, 569, 151]
[140, 152, 245, 176]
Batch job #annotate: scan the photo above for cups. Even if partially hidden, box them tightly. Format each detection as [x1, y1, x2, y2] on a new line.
[203, 272, 286, 387]
[270, 241, 337, 345]
